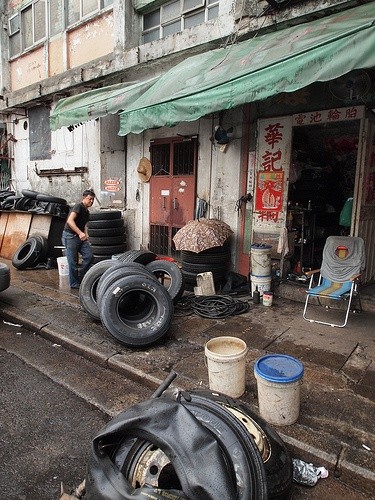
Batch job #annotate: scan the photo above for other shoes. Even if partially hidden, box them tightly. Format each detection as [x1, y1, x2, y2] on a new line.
[71, 284, 80, 289]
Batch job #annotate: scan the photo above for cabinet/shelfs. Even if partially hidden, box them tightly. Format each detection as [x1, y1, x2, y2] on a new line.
[286, 207, 314, 270]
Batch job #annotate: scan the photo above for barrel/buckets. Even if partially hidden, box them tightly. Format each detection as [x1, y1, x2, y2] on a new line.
[250, 244, 272, 276]
[57, 257, 70, 275]
[204, 336, 248, 401]
[250, 274, 272, 299]
[254, 354, 303, 427]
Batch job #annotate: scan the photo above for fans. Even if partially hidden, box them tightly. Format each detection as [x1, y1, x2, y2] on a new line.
[214, 123, 237, 144]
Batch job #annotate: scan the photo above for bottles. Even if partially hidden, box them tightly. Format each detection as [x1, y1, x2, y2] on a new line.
[253, 284, 260, 304]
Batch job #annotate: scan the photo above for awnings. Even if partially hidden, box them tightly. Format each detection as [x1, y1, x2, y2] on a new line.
[49, 74, 162, 130]
[117, 1, 375, 138]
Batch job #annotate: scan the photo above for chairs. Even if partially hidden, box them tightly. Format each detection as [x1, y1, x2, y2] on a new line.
[301, 235, 366, 328]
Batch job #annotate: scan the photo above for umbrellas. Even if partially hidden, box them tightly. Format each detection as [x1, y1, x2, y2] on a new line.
[171, 216, 235, 254]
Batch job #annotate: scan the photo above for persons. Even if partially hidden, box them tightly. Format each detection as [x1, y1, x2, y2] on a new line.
[338, 196, 354, 236]
[61, 190, 96, 289]
